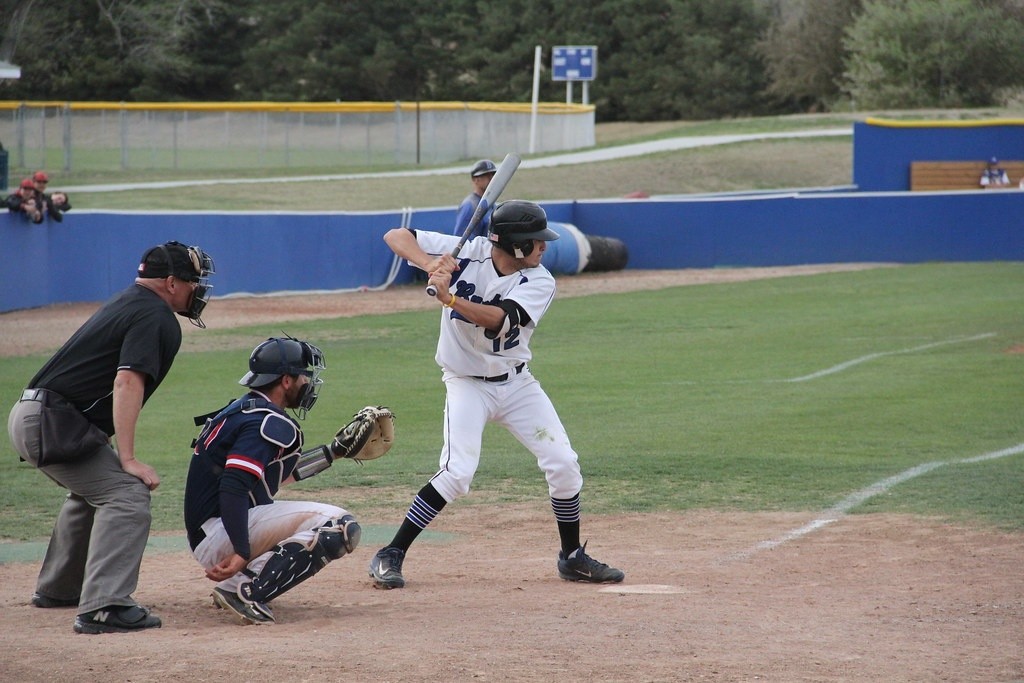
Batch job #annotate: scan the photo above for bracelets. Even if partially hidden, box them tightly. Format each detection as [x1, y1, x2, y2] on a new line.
[443, 293, 455, 308]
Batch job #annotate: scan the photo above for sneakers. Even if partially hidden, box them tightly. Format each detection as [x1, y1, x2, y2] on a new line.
[33, 593, 81, 608]
[558, 538, 625, 584]
[210, 587, 274, 625]
[74, 605, 162, 633]
[368, 548, 405, 588]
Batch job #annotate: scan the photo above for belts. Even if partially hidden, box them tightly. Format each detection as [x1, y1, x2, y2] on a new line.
[472, 362, 526, 381]
[190, 527, 207, 553]
[21, 389, 65, 404]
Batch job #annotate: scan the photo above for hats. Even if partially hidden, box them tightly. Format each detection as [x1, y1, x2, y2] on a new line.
[19, 178, 33, 189]
[34, 172, 48, 183]
[139, 244, 208, 282]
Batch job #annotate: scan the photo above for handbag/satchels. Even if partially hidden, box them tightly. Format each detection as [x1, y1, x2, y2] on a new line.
[38, 406, 107, 467]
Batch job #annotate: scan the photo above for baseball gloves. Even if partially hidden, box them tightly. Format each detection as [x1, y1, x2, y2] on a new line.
[331, 405, 394, 460]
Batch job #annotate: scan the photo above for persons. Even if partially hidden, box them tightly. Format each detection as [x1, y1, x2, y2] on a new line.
[370, 200, 625, 590]
[4, 171, 69, 222]
[7, 239, 215, 635]
[184, 337, 393, 623]
[980, 156, 1010, 188]
[454, 159, 496, 241]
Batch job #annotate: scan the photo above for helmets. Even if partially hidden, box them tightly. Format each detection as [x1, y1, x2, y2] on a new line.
[489, 201, 561, 248]
[239, 337, 309, 387]
[470, 160, 497, 176]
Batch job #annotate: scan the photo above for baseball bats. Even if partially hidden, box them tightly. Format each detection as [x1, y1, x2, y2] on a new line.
[427, 153, 522, 296]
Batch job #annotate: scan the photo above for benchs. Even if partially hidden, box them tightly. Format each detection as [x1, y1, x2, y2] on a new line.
[910, 161, 1024, 191]
